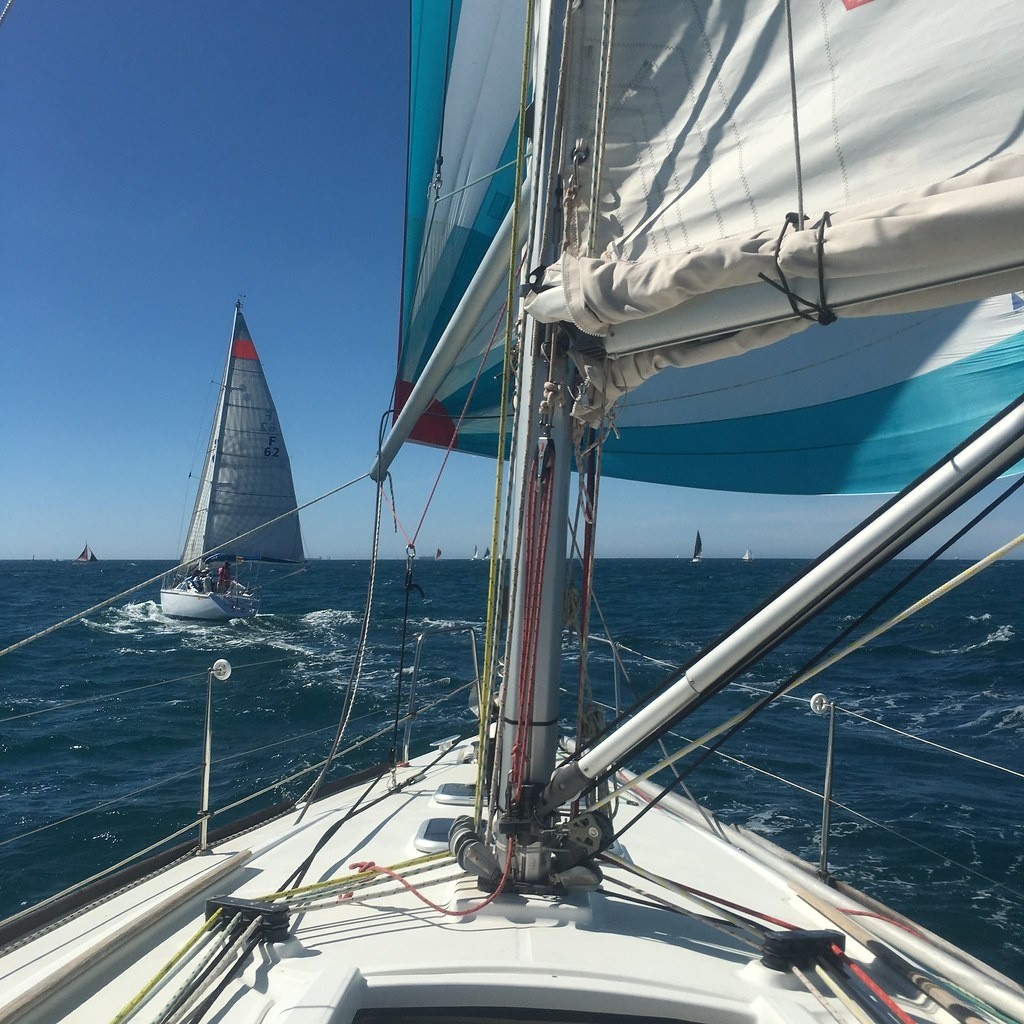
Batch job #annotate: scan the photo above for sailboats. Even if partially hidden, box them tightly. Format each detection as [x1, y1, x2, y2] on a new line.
[692, 528, 703, 563]
[469, 544, 478, 561]
[435, 548, 442, 562]
[481, 547, 491, 561]
[158, 298, 305, 617]
[3, 2, 1024, 1024]
[76, 540, 99, 565]
[741, 546, 755, 562]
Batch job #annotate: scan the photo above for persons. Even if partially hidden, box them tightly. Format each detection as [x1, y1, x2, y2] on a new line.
[216, 560, 232, 594]
[198, 569, 212, 592]
[189, 569, 200, 595]
